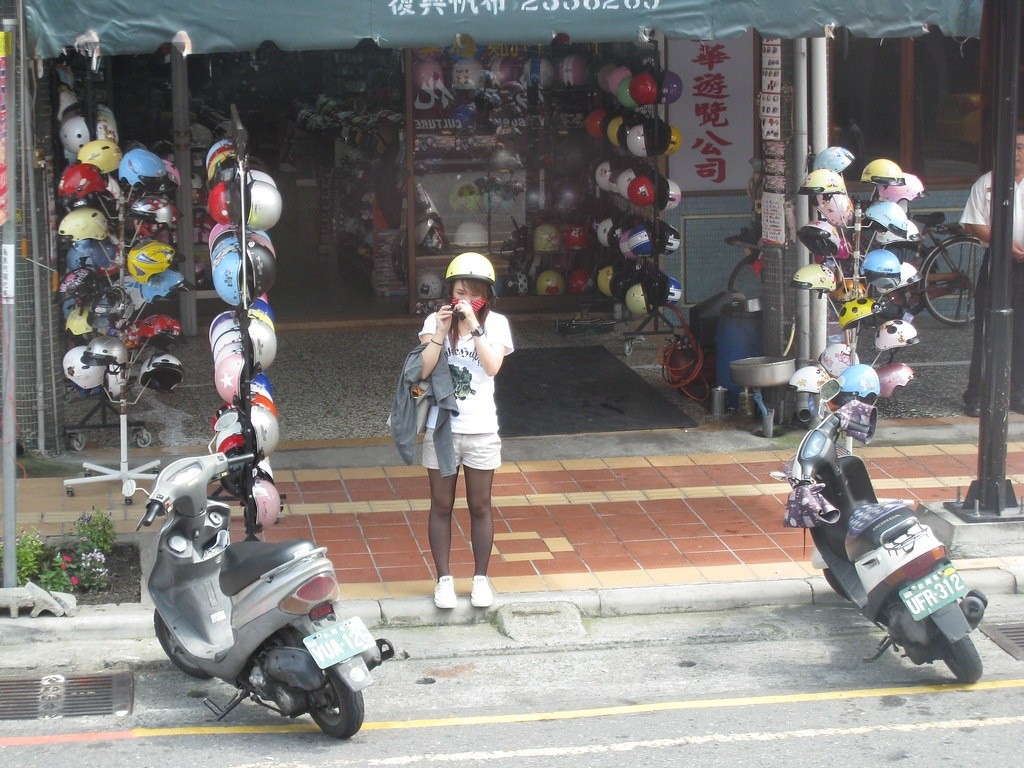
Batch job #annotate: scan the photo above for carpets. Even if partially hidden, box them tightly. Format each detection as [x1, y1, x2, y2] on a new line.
[494, 344, 699, 440]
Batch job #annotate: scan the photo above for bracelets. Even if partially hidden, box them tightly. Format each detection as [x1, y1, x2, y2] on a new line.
[431, 339, 442, 346]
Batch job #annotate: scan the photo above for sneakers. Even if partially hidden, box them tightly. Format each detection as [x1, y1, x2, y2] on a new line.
[433, 575, 457, 609]
[471, 576, 493, 607]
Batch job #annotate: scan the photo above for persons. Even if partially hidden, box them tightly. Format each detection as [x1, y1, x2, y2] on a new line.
[959, 129, 1024, 417]
[419, 253, 514, 608]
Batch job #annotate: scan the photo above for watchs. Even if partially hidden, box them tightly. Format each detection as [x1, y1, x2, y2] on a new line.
[471, 326, 484, 336]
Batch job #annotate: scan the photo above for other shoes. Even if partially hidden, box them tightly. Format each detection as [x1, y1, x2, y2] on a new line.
[1010, 399, 1024, 414]
[965, 400, 980, 417]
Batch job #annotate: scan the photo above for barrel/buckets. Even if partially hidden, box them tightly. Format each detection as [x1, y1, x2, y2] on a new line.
[716, 299, 764, 411]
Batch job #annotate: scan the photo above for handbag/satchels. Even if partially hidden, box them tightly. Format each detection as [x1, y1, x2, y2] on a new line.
[386, 399, 430, 435]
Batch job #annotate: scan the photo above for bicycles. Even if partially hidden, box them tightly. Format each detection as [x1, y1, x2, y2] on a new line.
[724, 212, 989, 327]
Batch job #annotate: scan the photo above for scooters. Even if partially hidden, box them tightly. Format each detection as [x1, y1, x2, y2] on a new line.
[770, 379, 987, 683]
[121, 413, 394, 739]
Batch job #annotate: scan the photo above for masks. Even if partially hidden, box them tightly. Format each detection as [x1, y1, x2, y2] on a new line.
[452, 297, 485, 312]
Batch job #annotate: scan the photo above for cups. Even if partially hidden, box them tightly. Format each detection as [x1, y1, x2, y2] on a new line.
[740, 297, 761, 312]
[711, 387, 729, 416]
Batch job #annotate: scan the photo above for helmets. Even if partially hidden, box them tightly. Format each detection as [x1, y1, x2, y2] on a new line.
[793, 145, 925, 398]
[445, 252, 495, 285]
[413, 55, 682, 315]
[51, 46, 406, 531]
